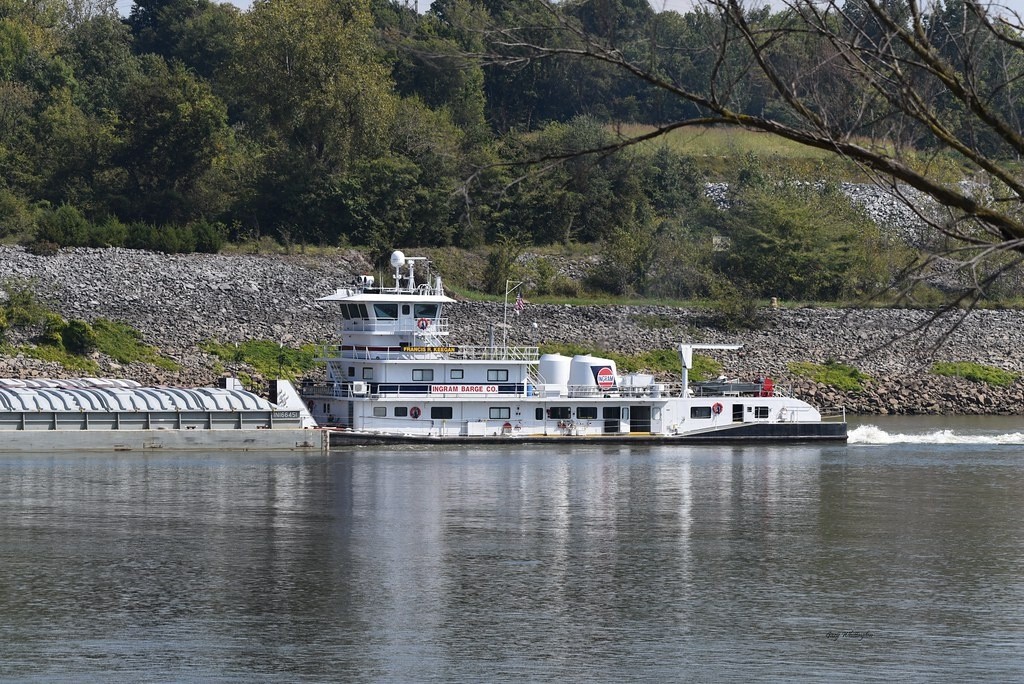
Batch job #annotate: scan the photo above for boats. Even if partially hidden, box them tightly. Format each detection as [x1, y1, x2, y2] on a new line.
[293, 250, 850, 446]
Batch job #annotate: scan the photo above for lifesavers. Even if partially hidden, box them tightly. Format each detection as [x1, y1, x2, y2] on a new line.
[411, 407, 421, 418]
[418, 318, 428, 329]
[713, 403, 723, 413]
